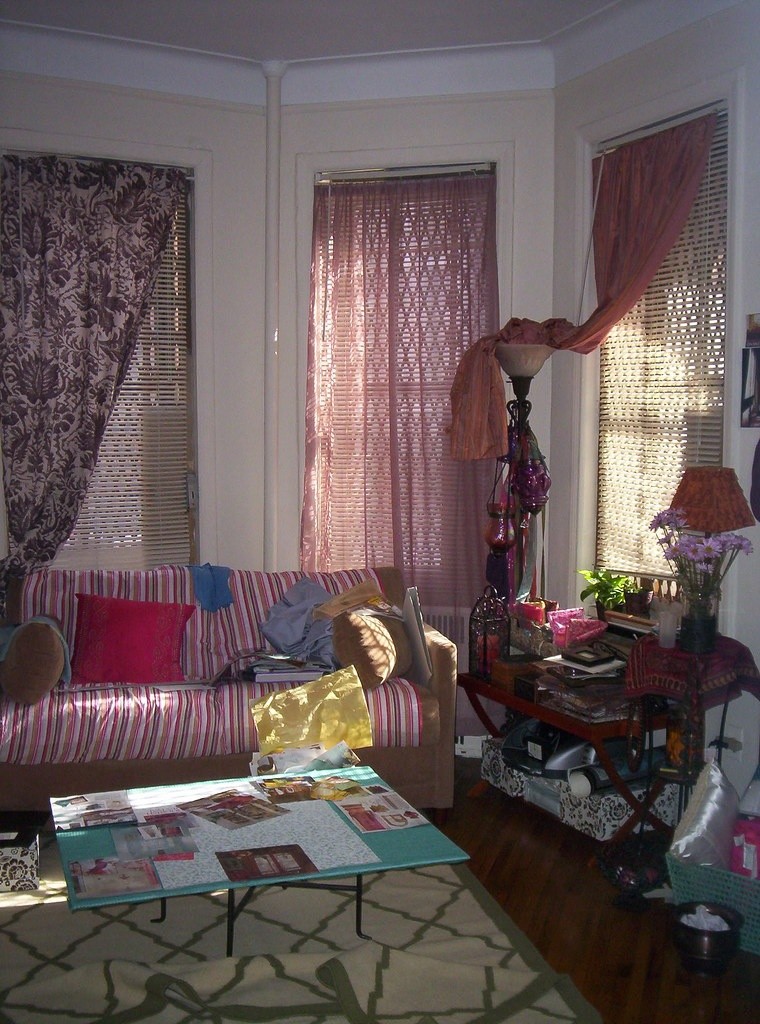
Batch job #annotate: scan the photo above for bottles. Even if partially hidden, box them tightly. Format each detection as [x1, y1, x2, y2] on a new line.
[659, 609, 677, 648]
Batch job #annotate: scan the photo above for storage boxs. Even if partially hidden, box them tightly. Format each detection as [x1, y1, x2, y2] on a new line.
[488, 654, 553, 704]
[0, 831, 40, 892]
[665, 849, 760, 956]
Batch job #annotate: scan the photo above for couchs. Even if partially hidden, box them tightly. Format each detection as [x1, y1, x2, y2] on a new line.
[0, 566, 456, 809]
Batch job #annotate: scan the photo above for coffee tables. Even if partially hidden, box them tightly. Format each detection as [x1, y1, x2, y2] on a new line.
[50, 767, 471, 960]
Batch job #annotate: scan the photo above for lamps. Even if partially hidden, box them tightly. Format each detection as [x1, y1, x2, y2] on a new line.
[667, 466, 756, 641]
[489, 345, 557, 597]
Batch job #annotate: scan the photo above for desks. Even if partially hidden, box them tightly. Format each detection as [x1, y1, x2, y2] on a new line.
[458, 652, 742, 860]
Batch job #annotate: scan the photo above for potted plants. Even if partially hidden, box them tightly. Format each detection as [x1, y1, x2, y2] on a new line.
[575, 567, 653, 624]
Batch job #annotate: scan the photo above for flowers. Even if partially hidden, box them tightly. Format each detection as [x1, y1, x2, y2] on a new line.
[649, 508, 755, 593]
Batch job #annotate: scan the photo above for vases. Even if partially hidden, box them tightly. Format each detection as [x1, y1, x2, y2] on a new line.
[684, 586, 721, 641]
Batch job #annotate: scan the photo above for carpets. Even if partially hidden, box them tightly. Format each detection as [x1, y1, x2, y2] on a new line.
[0, 827, 605, 1024]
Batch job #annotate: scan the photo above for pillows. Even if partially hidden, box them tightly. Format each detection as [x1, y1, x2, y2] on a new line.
[71, 593, 197, 683]
[332, 605, 413, 688]
[668, 756, 741, 872]
[3, 613, 66, 705]
